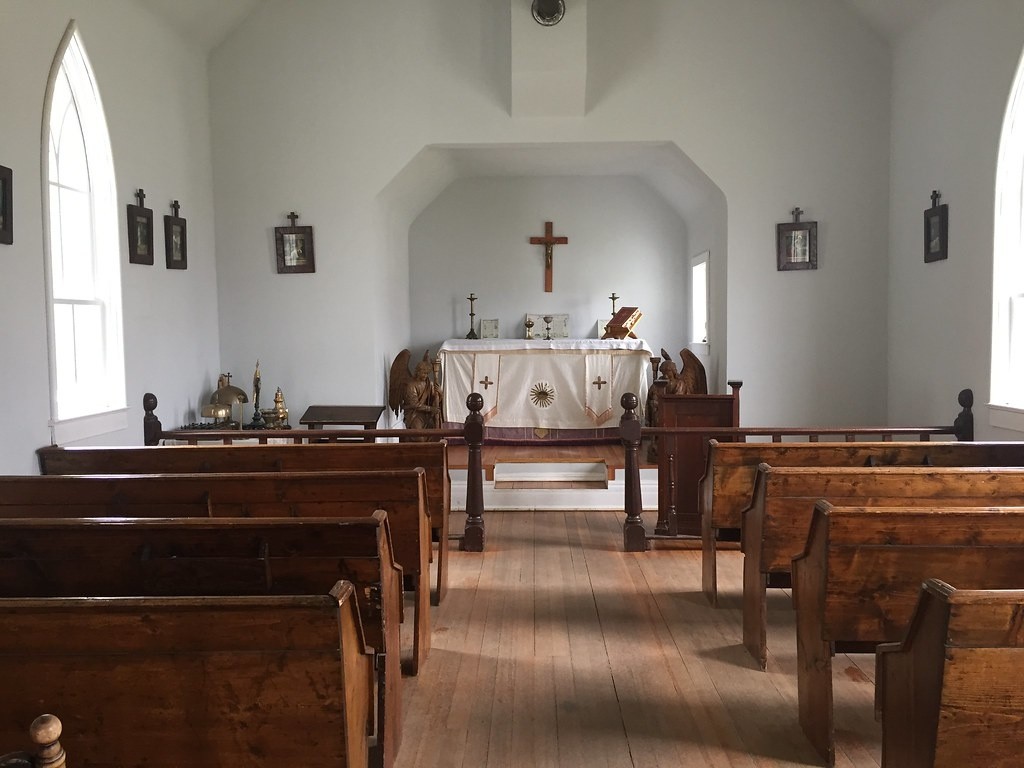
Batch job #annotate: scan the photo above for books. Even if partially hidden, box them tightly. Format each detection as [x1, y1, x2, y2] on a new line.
[525, 313, 570, 338]
[597, 319, 611, 338]
[480, 318, 499, 338]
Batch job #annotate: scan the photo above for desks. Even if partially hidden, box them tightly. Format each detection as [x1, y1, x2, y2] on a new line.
[438, 338, 653, 442]
[299, 405, 386, 443]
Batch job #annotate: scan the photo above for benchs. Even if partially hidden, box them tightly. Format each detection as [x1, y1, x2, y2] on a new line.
[0, 439, 452, 768]
[698, 439, 1024, 768]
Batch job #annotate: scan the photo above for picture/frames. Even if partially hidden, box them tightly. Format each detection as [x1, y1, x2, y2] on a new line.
[128, 204, 154, 265]
[165, 215, 187, 270]
[480, 318, 500, 339]
[597, 319, 611, 338]
[777, 221, 817, 271]
[924, 204, 948, 264]
[0, 165, 13, 245]
[275, 226, 315, 274]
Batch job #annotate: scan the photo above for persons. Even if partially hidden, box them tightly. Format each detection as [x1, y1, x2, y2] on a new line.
[646, 360, 687, 456]
[403, 360, 443, 442]
[541, 241, 558, 267]
